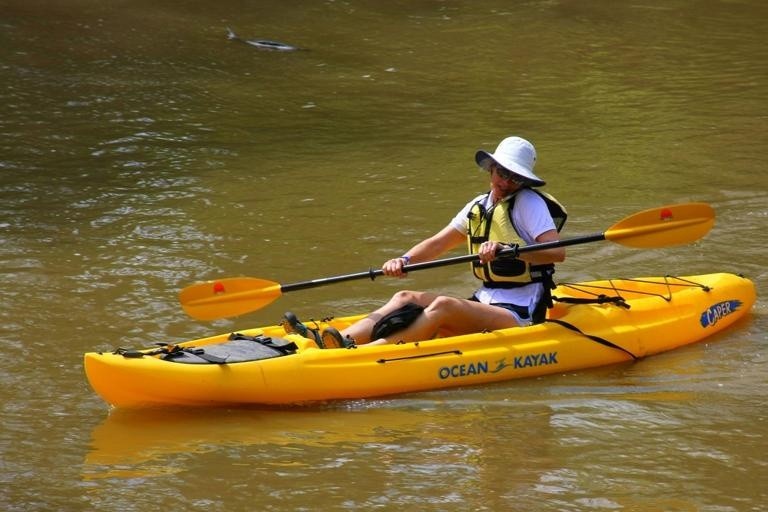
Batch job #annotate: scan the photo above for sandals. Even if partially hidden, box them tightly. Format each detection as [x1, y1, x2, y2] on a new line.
[283, 312, 344, 349]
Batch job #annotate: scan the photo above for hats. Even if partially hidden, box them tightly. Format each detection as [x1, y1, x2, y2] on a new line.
[475, 136, 545, 186]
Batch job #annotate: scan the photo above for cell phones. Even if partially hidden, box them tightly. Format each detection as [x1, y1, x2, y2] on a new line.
[501, 188, 523, 202]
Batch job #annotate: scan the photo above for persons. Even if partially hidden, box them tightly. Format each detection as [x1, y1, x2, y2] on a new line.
[284, 136, 564, 352]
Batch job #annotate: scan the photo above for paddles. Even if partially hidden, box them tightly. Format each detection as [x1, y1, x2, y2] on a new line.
[178, 204, 714, 319]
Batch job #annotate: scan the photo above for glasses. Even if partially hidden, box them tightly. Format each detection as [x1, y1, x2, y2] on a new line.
[496, 168, 525, 186]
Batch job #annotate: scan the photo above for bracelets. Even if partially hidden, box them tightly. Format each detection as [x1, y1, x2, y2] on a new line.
[397, 255, 412, 264]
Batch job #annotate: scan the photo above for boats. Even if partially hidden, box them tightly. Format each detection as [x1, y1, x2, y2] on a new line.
[81, 320, 754, 480]
[85, 269, 757, 405]
